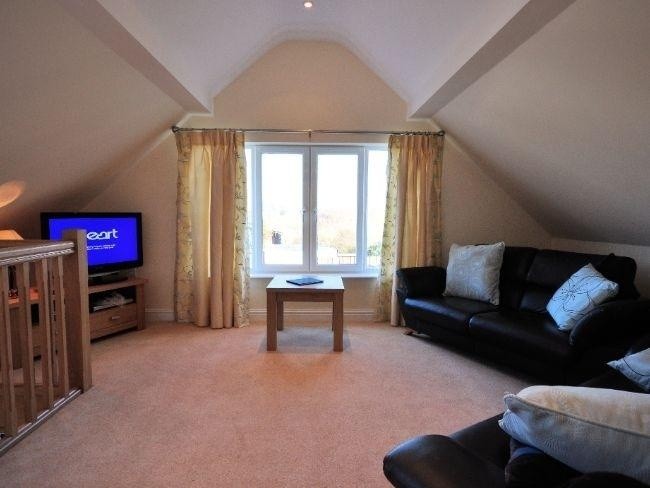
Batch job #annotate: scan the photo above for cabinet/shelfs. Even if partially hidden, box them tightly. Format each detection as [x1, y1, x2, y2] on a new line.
[8, 276, 149, 370]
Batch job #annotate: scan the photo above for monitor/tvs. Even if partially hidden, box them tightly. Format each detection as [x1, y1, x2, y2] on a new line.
[39, 210, 144, 286]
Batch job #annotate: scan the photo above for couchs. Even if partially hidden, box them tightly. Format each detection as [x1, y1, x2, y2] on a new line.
[382, 341, 650, 488]
[395, 242, 642, 386]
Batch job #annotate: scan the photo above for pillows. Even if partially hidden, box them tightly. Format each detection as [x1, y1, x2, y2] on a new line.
[607, 347, 650, 392]
[442, 241, 506, 306]
[546, 263, 619, 332]
[497, 386, 650, 486]
